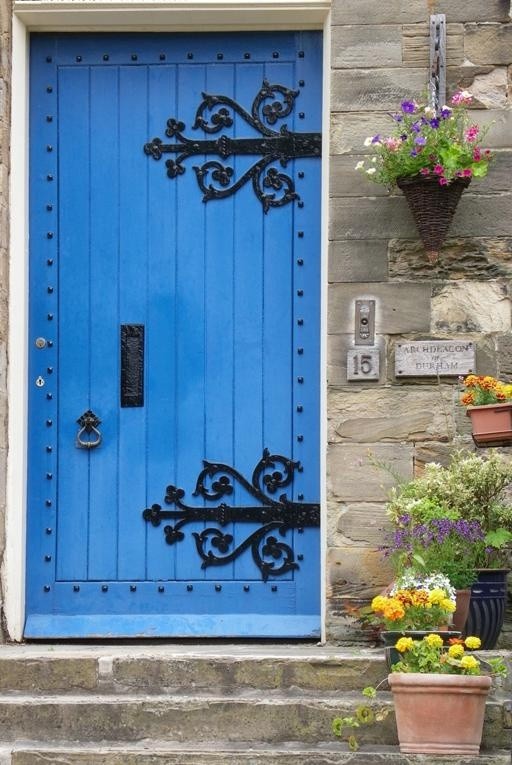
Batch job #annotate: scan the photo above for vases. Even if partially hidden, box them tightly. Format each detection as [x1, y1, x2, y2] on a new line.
[466, 403, 512, 448]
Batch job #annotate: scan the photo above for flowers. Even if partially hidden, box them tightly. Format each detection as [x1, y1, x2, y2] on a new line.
[460, 375, 512, 406]
[355, 85, 497, 196]
